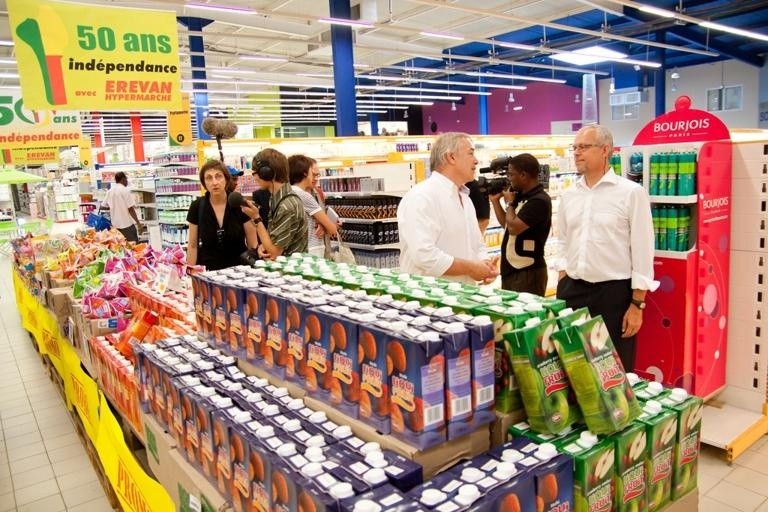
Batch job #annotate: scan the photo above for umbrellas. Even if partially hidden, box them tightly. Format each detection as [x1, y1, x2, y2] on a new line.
[0, 168, 51, 224]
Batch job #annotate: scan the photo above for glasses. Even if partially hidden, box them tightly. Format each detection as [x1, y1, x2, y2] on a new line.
[572, 143, 600, 152]
[216, 229, 225, 250]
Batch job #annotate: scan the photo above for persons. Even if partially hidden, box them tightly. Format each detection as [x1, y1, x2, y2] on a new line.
[395, 132, 501, 291]
[488, 153, 551, 298]
[550, 122, 660, 374]
[240, 148, 343, 262]
[465, 180, 489, 236]
[186, 158, 260, 271]
[98, 172, 143, 244]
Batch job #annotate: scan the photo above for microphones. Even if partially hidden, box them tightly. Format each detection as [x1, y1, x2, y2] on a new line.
[228, 192, 261, 209]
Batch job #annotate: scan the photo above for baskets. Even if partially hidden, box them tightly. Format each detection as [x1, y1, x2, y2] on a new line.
[86, 211, 111, 230]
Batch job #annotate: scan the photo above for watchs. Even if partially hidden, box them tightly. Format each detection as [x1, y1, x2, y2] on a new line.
[631, 299, 646, 309]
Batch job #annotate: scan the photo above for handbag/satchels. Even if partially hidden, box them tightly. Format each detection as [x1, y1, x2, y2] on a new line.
[240, 248, 257, 267]
[324, 230, 356, 264]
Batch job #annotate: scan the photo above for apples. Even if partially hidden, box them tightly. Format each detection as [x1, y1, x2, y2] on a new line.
[573, 442, 691, 512]
[531, 368, 630, 433]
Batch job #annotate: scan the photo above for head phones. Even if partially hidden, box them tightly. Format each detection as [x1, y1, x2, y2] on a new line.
[256, 152, 274, 181]
